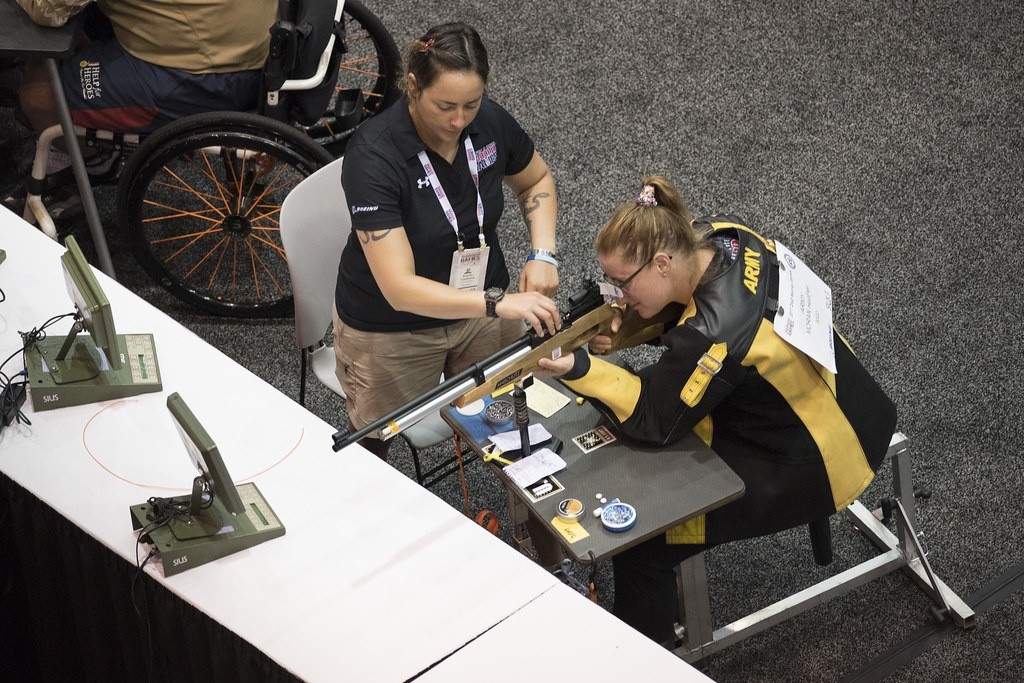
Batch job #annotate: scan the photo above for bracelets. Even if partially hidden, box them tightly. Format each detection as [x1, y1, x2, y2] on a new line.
[526, 250, 560, 269]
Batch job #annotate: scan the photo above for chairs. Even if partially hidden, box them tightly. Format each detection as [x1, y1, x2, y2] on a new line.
[279, 158, 477, 489]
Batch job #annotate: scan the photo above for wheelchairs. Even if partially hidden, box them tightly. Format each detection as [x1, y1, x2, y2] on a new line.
[0, 0, 409, 324]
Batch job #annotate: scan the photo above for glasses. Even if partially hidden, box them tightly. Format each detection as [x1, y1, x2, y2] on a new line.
[603, 256, 672, 289]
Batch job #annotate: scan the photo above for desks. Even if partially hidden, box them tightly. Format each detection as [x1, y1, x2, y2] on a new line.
[0, 0, 118, 282]
[409, 583, 717, 683]
[0, 203, 560, 683]
[440, 343, 745, 648]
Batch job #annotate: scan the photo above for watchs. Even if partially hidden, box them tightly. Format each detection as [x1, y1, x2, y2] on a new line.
[484, 286, 505, 318]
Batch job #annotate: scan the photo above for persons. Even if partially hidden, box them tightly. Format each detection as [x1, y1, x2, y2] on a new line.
[537, 174, 900, 649]
[329, 18, 563, 458]
[3, 0, 289, 213]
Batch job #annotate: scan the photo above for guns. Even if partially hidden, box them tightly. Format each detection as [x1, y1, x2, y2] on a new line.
[331, 276, 684, 453]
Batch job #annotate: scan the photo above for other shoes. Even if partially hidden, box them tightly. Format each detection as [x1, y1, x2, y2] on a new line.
[21, 144, 124, 190]
